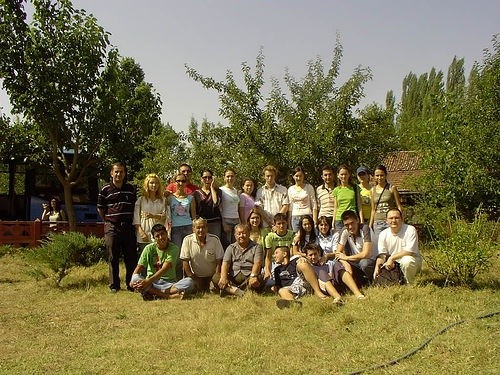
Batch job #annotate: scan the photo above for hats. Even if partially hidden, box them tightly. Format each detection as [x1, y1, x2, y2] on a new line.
[357, 167, 369, 175]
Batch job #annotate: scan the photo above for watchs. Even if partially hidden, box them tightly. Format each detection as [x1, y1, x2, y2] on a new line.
[249, 273, 258, 278]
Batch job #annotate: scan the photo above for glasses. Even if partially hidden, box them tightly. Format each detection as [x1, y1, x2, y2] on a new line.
[202, 176, 212, 180]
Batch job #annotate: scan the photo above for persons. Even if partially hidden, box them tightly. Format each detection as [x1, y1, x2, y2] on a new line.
[271, 246, 366, 310]
[180, 216, 224, 294]
[247, 211, 376, 295]
[373, 208, 422, 288]
[212, 224, 264, 298]
[42, 197, 67, 235]
[133, 164, 402, 282]
[129, 224, 194, 301]
[98, 162, 137, 294]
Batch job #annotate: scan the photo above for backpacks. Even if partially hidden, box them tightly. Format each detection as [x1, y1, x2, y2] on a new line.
[373, 261, 408, 288]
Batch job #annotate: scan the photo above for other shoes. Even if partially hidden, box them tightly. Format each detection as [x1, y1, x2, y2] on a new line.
[332, 297, 345, 306]
[276, 298, 302, 310]
[220, 288, 229, 297]
